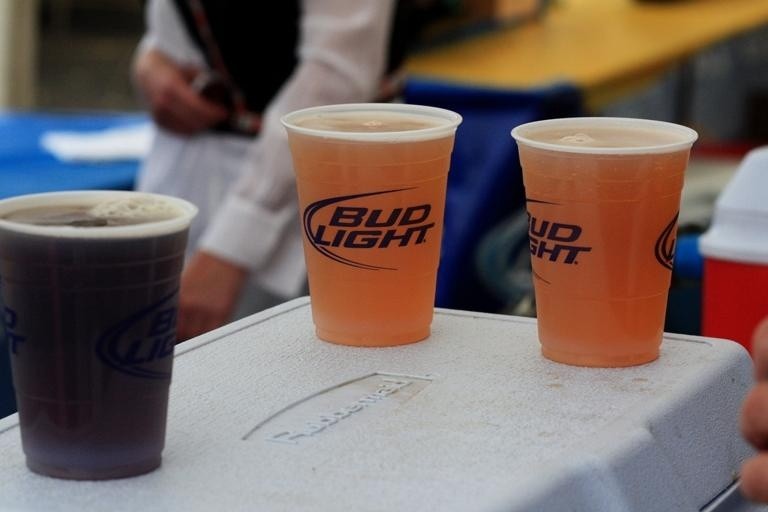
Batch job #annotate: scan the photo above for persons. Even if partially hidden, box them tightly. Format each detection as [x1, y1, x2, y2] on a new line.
[124, 1, 422, 341]
[733, 316, 767, 506]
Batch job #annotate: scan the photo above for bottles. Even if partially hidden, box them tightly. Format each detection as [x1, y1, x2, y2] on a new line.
[699, 143, 767, 373]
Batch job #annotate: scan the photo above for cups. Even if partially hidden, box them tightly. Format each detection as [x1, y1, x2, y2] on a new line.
[510, 118, 698, 369]
[0, 191, 199, 480]
[283, 100, 465, 348]
